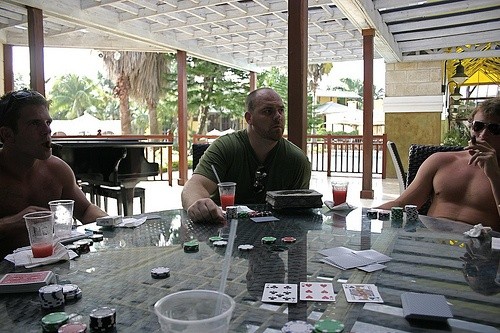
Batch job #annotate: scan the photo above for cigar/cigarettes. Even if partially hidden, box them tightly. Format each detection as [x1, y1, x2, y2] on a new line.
[472, 135, 476, 144]
[47, 143, 63, 151]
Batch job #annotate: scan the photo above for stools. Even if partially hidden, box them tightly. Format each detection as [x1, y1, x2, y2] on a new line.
[81, 183, 145, 216]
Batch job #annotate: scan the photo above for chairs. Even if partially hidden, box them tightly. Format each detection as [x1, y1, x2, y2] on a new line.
[405, 142, 465, 214]
[387, 140, 406, 193]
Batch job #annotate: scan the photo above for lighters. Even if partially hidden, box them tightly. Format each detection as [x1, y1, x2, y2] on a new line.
[84, 228, 103, 234]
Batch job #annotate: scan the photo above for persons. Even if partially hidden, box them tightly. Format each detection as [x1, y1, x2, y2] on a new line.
[0, 88, 110, 263]
[182, 87, 312, 224]
[372, 96, 500, 233]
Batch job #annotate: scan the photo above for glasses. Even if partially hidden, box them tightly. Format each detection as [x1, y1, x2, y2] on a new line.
[0, 91, 45, 124]
[472, 120, 500, 136]
[465, 266, 495, 277]
[253, 165, 267, 192]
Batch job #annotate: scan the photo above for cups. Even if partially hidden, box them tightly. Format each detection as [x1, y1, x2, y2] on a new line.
[47, 200, 75, 239]
[23, 211, 56, 258]
[154, 289, 235, 333]
[331, 181, 349, 208]
[217, 182, 236, 210]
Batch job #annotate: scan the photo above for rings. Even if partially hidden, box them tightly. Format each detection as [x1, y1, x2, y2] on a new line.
[479, 152, 482, 156]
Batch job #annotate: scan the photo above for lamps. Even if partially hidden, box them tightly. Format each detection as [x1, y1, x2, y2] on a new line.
[442, 59, 469, 121]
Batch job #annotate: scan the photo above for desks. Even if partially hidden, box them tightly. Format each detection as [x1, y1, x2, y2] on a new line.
[0, 205, 500, 333]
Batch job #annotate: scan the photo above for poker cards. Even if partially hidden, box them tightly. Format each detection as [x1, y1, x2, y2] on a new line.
[341, 283, 384, 303]
[261, 282, 298, 303]
[0, 271, 57, 294]
[299, 281, 336, 302]
[250, 216, 280, 223]
[315, 246, 394, 273]
[399, 290, 454, 321]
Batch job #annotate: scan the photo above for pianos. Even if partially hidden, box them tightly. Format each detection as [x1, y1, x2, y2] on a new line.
[51, 143, 160, 218]
